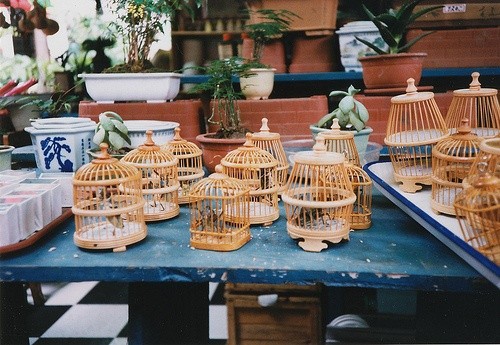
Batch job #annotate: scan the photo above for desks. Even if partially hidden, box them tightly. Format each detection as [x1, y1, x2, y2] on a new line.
[0, 176, 500, 345]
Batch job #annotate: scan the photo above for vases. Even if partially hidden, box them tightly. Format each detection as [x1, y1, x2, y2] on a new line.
[0, 145, 15, 172]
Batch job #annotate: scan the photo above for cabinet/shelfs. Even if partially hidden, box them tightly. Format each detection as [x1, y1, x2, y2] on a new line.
[170, 0, 500, 84]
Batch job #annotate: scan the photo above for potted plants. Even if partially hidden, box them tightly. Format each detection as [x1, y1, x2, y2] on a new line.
[176, 57, 270, 173]
[234, 9, 303, 101]
[351, 0, 445, 89]
[77, 0, 203, 104]
[52, 14, 118, 92]
[86, 110, 143, 198]
[310, 85, 374, 165]
[336, 6, 361, 28]
